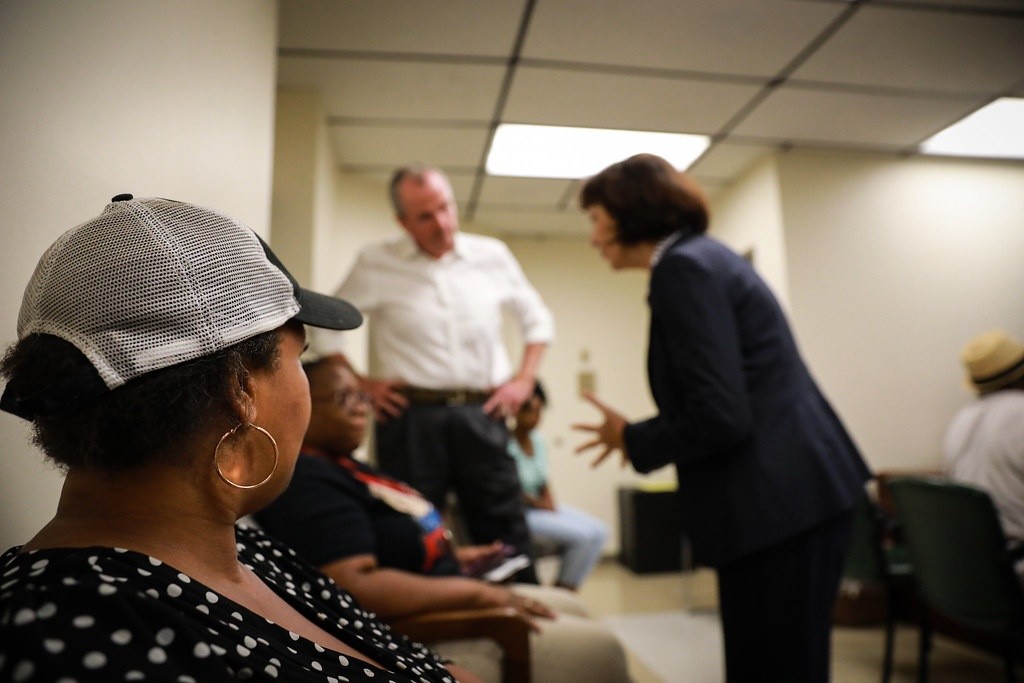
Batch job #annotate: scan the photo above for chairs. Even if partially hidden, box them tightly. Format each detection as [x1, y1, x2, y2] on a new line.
[876, 471, 1024, 683]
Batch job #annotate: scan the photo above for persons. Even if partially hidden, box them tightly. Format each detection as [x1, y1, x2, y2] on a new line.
[312, 167, 557, 586]
[249, 353, 630, 683]
[0, 198, 491, 683]
[946, 328, 1023, 581]
[571, 154, 872, 683]
[505, 376, 608, 594]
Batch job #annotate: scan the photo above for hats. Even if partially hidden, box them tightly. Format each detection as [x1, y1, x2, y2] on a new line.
[960, 331, 1023, 386]
[0, 195, 361, 421]
